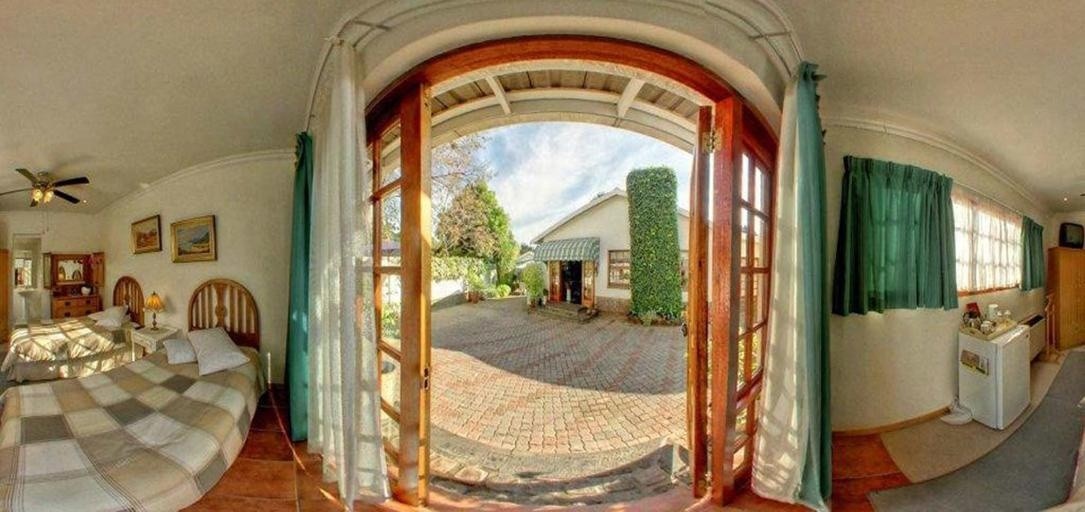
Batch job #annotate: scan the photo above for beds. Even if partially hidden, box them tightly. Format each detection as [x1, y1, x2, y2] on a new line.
[0, 277, 269, 511]
[1, 275, 146, 386]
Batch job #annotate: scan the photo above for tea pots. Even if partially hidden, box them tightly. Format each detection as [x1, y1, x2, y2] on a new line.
[964, 311, 980, 326]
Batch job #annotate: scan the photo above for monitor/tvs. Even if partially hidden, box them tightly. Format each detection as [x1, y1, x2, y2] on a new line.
[1059, 222, 1084, 248]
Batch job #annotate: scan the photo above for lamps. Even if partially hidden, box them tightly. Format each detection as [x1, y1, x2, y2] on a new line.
[30, 187, 55, 234]
[141, 289, 167, 331]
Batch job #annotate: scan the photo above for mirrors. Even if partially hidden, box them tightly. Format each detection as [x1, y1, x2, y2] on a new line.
[13, 256, 33, 288]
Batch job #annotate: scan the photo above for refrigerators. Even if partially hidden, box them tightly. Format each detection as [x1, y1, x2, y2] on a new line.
[958, 315, 1032, 431]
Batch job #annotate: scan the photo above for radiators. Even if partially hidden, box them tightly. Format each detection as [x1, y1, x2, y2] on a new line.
[1017, 312, 1046, 363]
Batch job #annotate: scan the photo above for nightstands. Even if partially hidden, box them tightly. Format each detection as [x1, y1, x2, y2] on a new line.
[129, 322, 180, 362]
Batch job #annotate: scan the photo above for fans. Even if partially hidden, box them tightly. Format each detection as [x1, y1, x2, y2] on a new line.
[0, 167, 89, 209]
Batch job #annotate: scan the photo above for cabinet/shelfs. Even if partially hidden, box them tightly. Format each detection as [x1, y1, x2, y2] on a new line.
[42, 251, 106, 319]
[0, 248, 13, 348]
[1046, 245, 1085, 350]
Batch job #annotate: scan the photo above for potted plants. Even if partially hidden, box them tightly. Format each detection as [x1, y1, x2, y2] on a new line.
[521, 261, 547, 313]
[464, 255, 487, 305]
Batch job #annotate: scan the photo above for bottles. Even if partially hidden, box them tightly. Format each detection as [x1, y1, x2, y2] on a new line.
[981, 304, 1013, 332]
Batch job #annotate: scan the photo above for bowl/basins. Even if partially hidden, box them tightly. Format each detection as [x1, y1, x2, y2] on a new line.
[81, 287, 91, 294]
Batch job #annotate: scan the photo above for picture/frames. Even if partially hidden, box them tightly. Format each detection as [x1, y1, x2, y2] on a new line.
[169, 213, 218, 265]
[130, 213, 163, 255]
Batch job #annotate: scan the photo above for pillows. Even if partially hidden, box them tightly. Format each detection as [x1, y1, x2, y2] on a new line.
[185, 326, 252, 378]
[161, 338, 197, 365]
[93, 304, 130, 331]
[87, 311, 131, 325]
